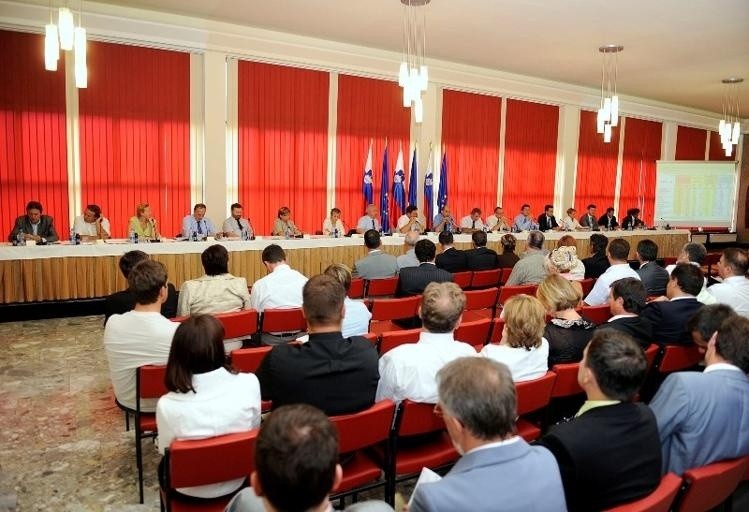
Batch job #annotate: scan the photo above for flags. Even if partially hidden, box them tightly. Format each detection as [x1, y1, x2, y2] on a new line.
[437, 141, 448, 213]
[408, 141, 418, 208]
[363, 138, 374, 204]
[381, 136, 389, 234]
[423, 140, 434, 231]
[393, 140, 405, 213]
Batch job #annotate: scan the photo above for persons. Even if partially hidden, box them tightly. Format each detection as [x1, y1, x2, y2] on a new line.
[287, 263, 372, 345]
[393, 239, 451, 328]
[644, 263, 703, 332]
[537, 205, 559, 232]
[434, 231, 472, 273]
[595, 277, 659, 348]
[497, 234, 520, 269]
[350, 230, 396, 280]
[583, 240, 641, 307]
[395, 231, 436, 275]
[685, 304, 739, 355]
[255, 274, 379, 417]
[707, 248, 748, 317]
[433, 206, 456, 232]
[402, 356, 566, 511]
[536, 274, 599, 365]
[622, 208, 643, 231]
[177, 245, 252, 355]
[479, 294, 548, 382]
[357, 206, 380, 234]
[531, 327, 662, 511]
[459, 208, 483, 232]
[223, 203, 254, 237]
[581, 234, 611, 278]
[579, 204, 599, 227]
[104, 260, 182, 417]
[549, 235, 585, 281]
[513, 204, 536, 231]
[635, 240, 670, 296]
[272, 207, 301, 236]
[182, 203, 216, 239]
[463, 231, 499, 270]
[250, 245, 309, 345]
[396, 205, 424, 233]
[8, 200, 59, 246]
[128, 202, 161, 240]
[72, 205, 111, 239]
[561, 208, 583, 231]
[598, 208, 616, 230]
[103, 250, 176, 328]
[504, 231, 552, 287]
[648, 317, 749, 477]
[665, 242, 708, 292]
[222, 404, 394, 512]
[156, 314, 261, 505]
[376, 281, 481, 403]
[323, 208, 345, 236]
[486, 207, 511, 231]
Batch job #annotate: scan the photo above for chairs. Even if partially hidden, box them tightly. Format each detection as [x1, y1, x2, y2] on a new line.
[134, 366, 170, 504]
[659, 345, 701, 372]
[362, 332, 377, 345]
[671, 456, 749, 512]
[606, 471, 683, 512]
[212, 308, 259, 350]
[552, 362, 584, 398]
[497, 285, 537, 316]
[487, 317, 506, 343]
[159, 427, 261, 512]
[582, 304, 610, 324]
[644, 342, 659, 369]
[230, 345, 275, 413]
[325, 399, 396, 511]
[258, 307, 307, 344]
[364, 399, 459, 510]
[379, 328, 422, 356]
[461, 287, 499, 321]
[365, 277, 398, 298]
[452, 271, 472, 290]
[544, 306, 582, 324]
[455, 317, 492, 353]
[499, 267, 512, 286]
[367, 295, 423, 332]
[579, 278, 593, 297]
[471, 268, 501, 288]
[514, 371, 557, 444]
[348, 279, 365, 300]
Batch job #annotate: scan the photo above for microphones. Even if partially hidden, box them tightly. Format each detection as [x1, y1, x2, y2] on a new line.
[204, 220, 208, 240]
[99, 218, 103, 240]
[660, 218, 664, 228]
[247, 218, 255, 240]
[150, 219, 160, 242]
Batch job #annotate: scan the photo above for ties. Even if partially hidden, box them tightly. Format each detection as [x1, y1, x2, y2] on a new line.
[197, 221, 203, 234]
[497, 218, 500, 231]
[372, 219, 376, 230]
[472, 221, 475, 229]
[444, 222, 448, 232]
[523, 218, 526, 224]
[548, 218, 550, 228]
[235, 218, 243, 231]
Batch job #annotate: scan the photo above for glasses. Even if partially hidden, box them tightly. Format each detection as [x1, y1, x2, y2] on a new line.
[434, 400, 453, 418]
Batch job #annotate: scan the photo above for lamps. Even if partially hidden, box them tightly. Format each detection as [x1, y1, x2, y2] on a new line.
[604, 124, 611, 143]
[611, 95, 619, 128]
[75, 26, 87, 89]
[58, 7, 75, 51]
[43, 23, 60, 72]
[604, 98, 611, 121]
[718, 119, 740, 156]
[398, 62, 428, 123]
[597, 109, 604, 133]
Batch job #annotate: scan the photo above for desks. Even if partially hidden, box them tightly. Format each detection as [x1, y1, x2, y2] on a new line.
[1, 228, 692, 304]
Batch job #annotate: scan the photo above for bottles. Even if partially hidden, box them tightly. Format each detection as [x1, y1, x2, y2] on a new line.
[129, 227, 136, 242]
[188, 226, 193, 241]
[18, 228, 25, 246]
[70, 228, 75, 242]
